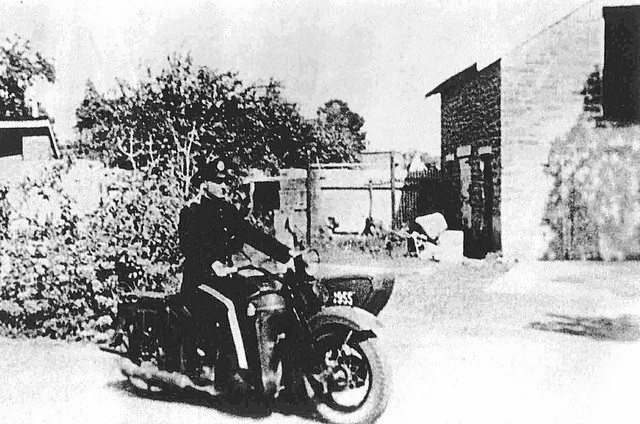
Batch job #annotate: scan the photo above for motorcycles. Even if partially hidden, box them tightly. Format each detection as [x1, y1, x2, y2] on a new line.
[100, 217, 394, 424]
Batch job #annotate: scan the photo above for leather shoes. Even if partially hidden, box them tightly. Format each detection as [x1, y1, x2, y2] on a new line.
[215, 372, 256, 391]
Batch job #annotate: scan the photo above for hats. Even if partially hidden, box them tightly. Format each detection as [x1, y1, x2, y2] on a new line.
[190, 155, 234, 189]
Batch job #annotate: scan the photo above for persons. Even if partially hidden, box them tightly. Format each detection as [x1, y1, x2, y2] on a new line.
[178, 157, 311, 417]
